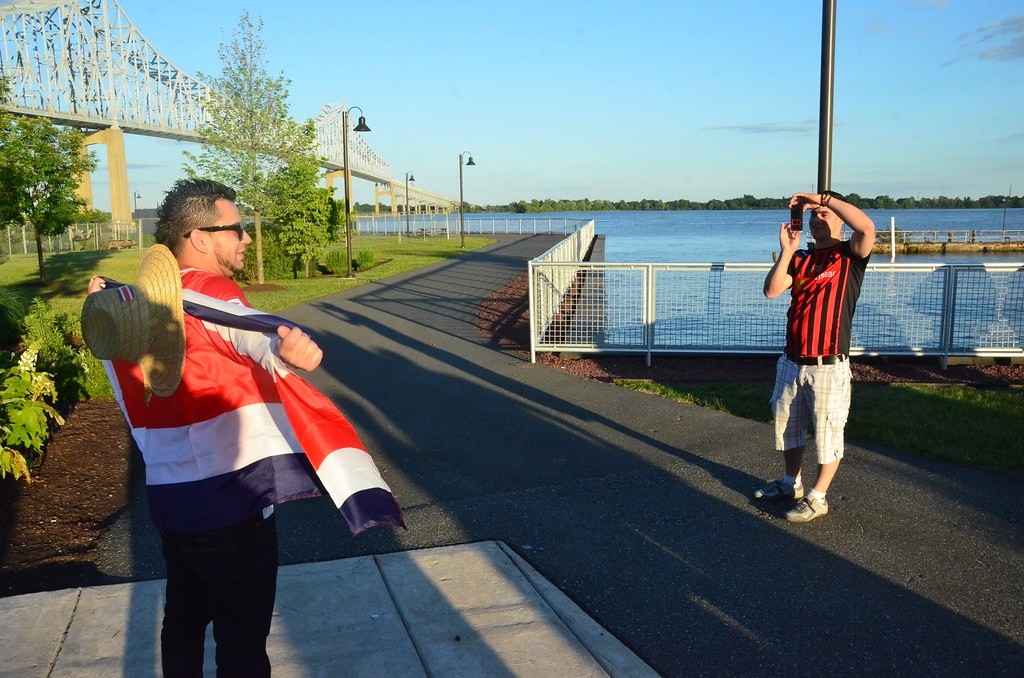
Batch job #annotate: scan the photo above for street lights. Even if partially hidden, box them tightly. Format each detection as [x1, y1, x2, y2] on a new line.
[405, 170, 417, 238]
[342, 106, 372, 277]
[459, 150, 476, 248]
[134, 191, 142, 219]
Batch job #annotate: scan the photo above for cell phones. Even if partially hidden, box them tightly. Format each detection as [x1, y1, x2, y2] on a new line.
[791, 201, 804, 231]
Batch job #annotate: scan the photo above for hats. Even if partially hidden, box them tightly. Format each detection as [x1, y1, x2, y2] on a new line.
[81, 244, 186, 396]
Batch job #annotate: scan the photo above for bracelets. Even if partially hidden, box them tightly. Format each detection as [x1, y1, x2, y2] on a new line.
[820, 193, 831, 207]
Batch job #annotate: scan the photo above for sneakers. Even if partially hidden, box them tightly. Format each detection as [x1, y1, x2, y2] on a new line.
[754, 478, 803, 498]
[785, 494, 829, 521]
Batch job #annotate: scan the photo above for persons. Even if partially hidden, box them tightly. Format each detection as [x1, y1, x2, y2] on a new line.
[753, 190, 876, 522]
[87, 178, 324, 678]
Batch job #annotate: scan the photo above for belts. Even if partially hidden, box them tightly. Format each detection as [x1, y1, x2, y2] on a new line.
[784, 353, 847, 365]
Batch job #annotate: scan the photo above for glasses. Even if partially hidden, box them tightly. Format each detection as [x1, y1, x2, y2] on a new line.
[183, 222, 244, 241]
